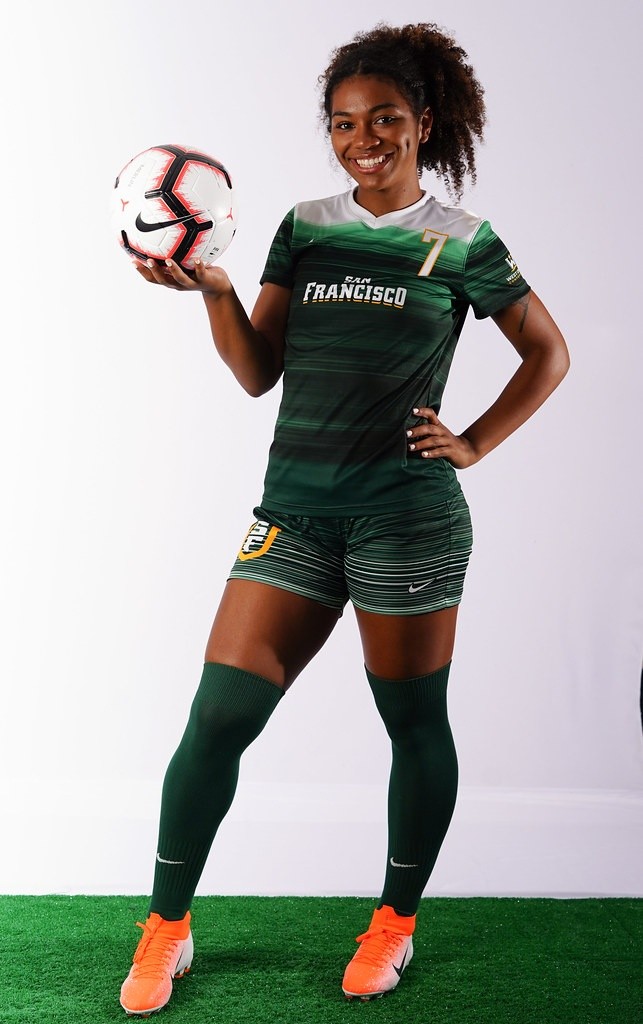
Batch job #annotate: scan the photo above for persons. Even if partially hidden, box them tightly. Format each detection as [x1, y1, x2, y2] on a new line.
[120, 21, 572, 1018]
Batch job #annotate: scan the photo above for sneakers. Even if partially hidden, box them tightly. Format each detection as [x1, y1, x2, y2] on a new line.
[341, 905, 415, 1001]
[120, 911, 194, 1015]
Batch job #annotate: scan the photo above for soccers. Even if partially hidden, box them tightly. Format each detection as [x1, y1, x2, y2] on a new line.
[114, 145, 238, 272]
